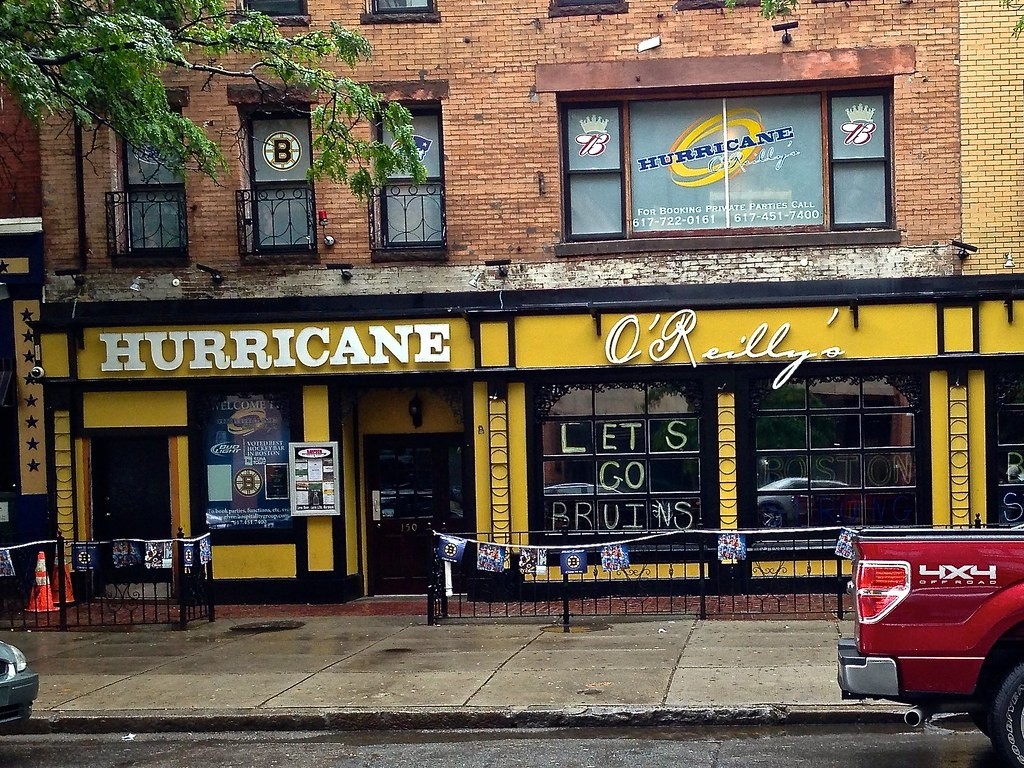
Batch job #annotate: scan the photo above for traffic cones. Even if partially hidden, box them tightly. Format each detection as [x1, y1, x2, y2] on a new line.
[51, 544, 76, 604]
[24, 551, 60, 613]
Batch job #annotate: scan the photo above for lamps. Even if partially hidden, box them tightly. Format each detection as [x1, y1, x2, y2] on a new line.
[129, 270, 154, 292]
[1004, 251, 1015, 267]
[319, 210, 336, 246]
[468, 269, 484, 288]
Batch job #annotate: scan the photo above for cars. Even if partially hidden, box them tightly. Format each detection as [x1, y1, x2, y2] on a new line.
[757, 478, 850, 528]
[836, 526, 1024, 768]
[0, 641, 39, 727]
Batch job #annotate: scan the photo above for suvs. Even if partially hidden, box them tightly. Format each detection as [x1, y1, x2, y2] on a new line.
[544, 483, 624, 527]
